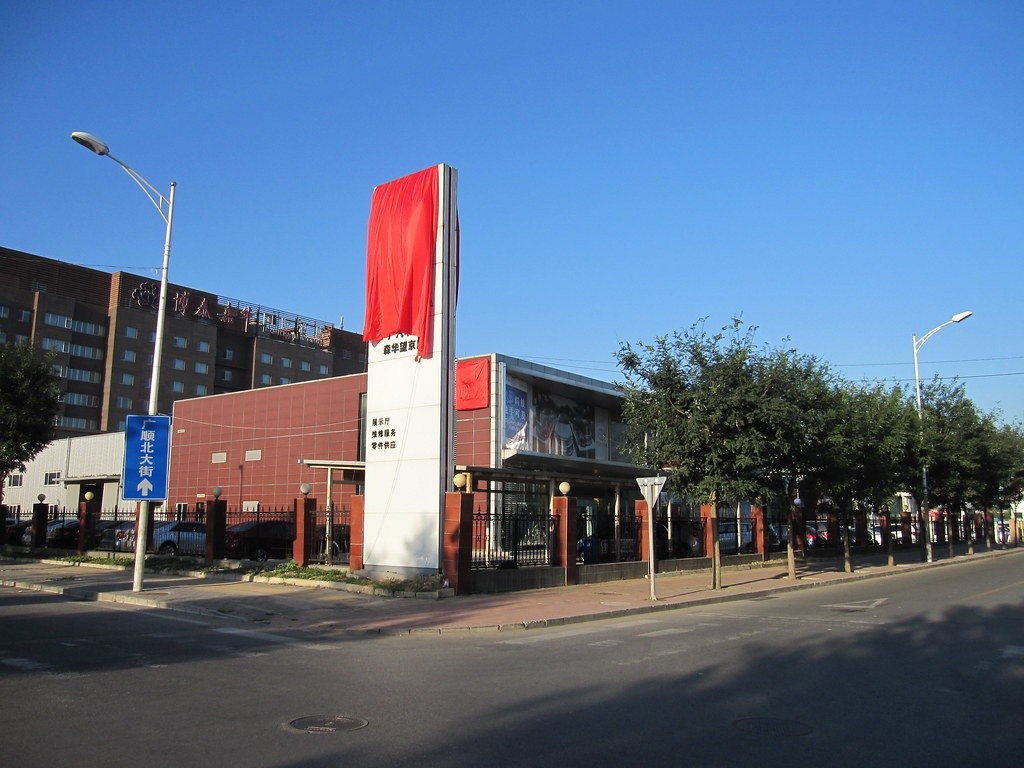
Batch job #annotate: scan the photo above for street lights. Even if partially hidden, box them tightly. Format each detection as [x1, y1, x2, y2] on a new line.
[913, 311, 975, 564]
[72, 129, 177, 592]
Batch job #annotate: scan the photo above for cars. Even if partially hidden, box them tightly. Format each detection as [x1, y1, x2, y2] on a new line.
[224, 520, 340, 564]
[573, 521, 920, 566]
[5, 517, 165, 552]
[148, 521, 210, 556]
